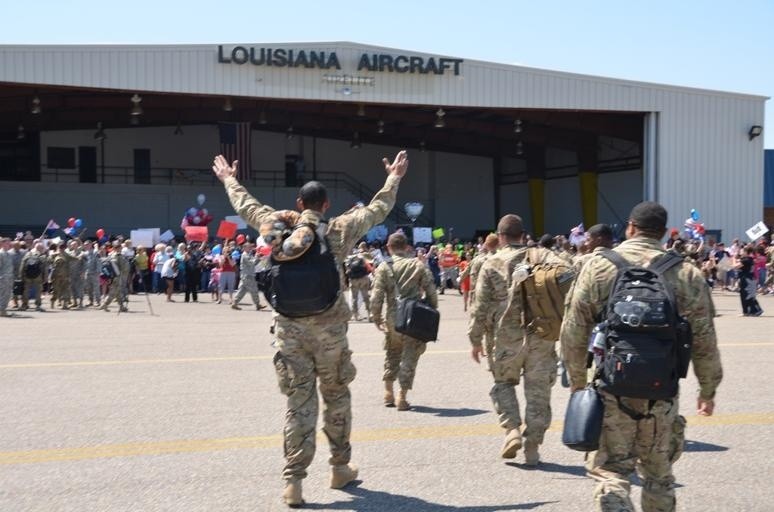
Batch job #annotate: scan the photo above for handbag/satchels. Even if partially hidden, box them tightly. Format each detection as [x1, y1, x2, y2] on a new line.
[563, 389, 603, 451]
[395, 298, 439, 342]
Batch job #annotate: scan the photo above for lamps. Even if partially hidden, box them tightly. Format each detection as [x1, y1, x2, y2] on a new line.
[258, 107, 268, 126]
[376, 120, 386, 135]
[129, 117, 142, 125]
[418, 138, 426, 153]
[357, 104, 367, 117]
[286, 130, 295, 142]
[516, 142, 523, 154]
[350, 132, 361, 150]
[131, 96, 144, 116]
[30, 95, 43, 114]
[223, 98, 233, 112]
[17, 125, 25, 140]
[434, 110, 446, 128]
[513, 119, 522, 134]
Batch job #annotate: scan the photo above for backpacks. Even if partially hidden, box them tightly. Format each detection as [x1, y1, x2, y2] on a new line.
[24, 251, 43, 278]
[268, 216, 339, 317]
[346, 256, 367, 278]
[521, 248, 576, 340]
[594, 246, 691, 400]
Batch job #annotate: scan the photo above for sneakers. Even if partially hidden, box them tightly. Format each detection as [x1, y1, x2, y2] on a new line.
[525, 448, 539, 466]
[232, 304, 240, 310]
[257, 304, 267, 310]
[330, 463, 358, 488]
[280, 480, 301, 504]
[502, 428, 521, 458]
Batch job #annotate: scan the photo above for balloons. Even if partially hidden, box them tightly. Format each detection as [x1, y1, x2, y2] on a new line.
[64, 217, 106, 240]
[185, 192, 212, 226]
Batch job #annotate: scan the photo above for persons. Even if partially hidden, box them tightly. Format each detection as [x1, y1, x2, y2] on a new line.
[464, 200, 722, 512]
[212, 151, 409, 506]
[342, 233, 502, 320]
[663, 229, 773, 317]
[0, 230, 271, 317]
[370, 234, 439, 410]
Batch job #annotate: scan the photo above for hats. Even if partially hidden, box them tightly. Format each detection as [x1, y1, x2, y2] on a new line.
[272, 226, 315, 261]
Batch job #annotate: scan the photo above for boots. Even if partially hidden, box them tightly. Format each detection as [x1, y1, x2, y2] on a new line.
[51, 298, 127, 311]
[385, 381, 394, 403]
[397, 390, 409, 410]
